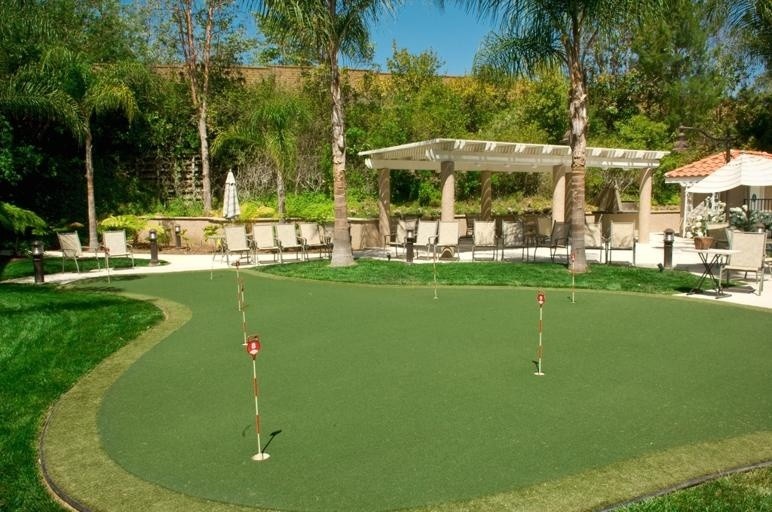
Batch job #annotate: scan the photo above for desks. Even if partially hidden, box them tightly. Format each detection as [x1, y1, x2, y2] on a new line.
[208, 234, 252, 261]
[680, 247, 741, 299]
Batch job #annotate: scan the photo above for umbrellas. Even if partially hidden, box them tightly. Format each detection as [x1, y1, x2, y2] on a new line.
[222, 169, 241, 224]
[683, 152, 772, 231]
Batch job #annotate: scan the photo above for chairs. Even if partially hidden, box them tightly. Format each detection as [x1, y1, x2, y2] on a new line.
[56, 230, 101, 272]
[725, 227, 772, 279]
[384, 215, 639, 265]
[349, 224, 365, 255]
[224, 223, 333, 266]
[103, 229, 134, 272]
[718, 232, 767, 296]
